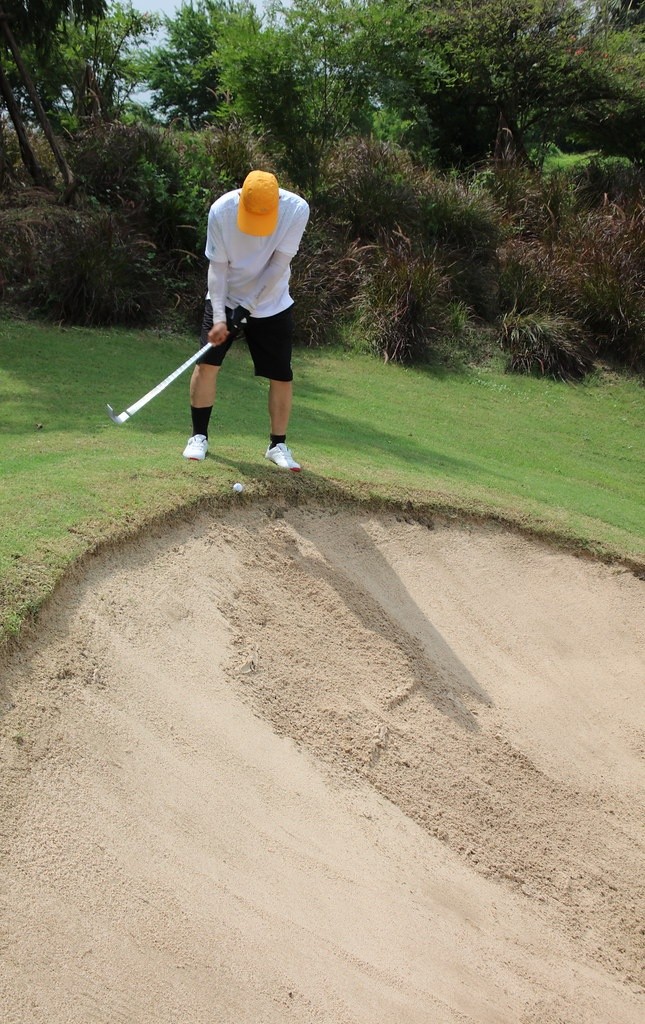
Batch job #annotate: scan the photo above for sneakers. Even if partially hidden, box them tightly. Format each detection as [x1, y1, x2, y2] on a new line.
[183, 433, 210, 461]
[265, 442, 301, 473]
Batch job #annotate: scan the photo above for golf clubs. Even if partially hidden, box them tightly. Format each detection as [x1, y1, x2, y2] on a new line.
[105, 311, 247, 426]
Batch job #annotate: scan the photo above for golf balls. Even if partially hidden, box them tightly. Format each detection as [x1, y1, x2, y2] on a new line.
[233, 483, 243, 492]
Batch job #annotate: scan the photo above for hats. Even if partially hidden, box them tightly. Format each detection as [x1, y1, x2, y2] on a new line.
[237, 170, 279, 237]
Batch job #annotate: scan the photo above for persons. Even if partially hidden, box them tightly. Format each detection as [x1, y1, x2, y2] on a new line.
[182, 170, 311, 472]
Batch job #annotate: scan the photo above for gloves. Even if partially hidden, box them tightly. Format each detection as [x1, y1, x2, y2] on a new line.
[229, 297, 256, 339]
